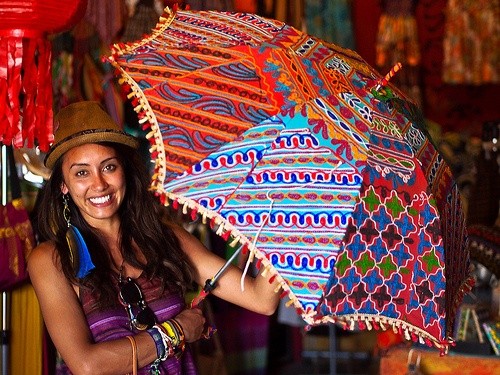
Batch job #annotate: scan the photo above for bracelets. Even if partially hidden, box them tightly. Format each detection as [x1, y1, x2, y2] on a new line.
[146, 318, 187, 375]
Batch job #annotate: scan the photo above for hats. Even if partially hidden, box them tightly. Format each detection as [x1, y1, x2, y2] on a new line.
[43, 100, 142, 170]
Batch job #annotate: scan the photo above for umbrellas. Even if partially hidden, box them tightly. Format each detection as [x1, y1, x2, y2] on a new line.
[99, 4, 473, 359]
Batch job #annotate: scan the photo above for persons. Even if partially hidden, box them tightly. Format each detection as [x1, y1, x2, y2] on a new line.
[28, 99, 284, 375]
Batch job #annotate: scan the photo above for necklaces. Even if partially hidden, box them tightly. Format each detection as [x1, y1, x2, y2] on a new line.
[102, 234, 132, 286]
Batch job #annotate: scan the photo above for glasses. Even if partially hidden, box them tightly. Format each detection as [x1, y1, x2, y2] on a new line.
[120, 275, 155, 327]
